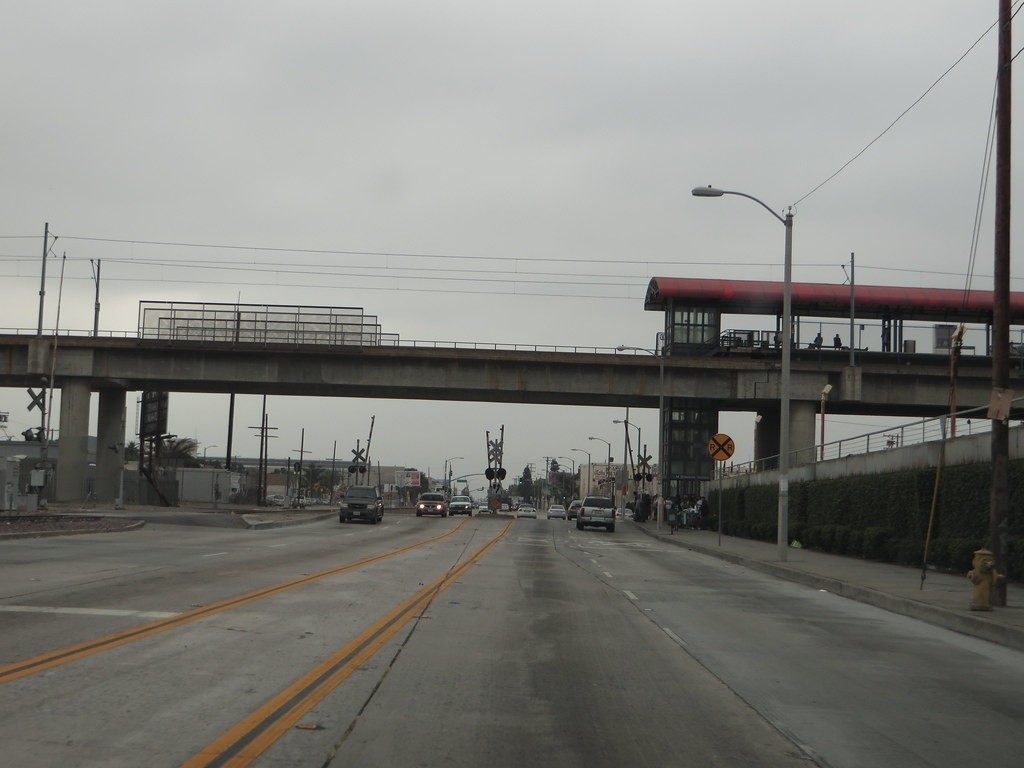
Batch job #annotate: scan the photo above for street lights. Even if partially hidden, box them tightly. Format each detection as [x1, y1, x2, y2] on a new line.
[444, 455, 465, 492]
[588, 437, 611, 496]
[558, 457, 574, 500]
[554, 464, 572, 501]
[613, 420, 641, 492]
[571, 448, 591, 496]
[690, 185, 794, 562]
[616, 344, 665, 532]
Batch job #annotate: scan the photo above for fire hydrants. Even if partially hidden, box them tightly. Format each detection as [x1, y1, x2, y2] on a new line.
[967, 547, 1005, 612]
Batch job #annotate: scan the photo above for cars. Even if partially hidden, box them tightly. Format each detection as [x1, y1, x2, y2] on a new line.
[566, 500, 582, 521]
[498, 503, 518, 512]
[547, 505, 566, 520]
[479, 502, 492, 514]
[517, 504, 537, 519]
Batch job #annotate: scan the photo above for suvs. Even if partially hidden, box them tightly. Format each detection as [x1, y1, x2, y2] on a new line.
[575, 495, 617, 532]
[338, 484, 384, 525]
[416, 492, 448, 518]
[447, 495, 474, 516]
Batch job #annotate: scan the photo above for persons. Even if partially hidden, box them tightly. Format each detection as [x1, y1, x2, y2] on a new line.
[774, 331, 780, 348]
[650, 493, 708, 531]
[809, 333, 823, 349]
[833, 334, 842, 350]
